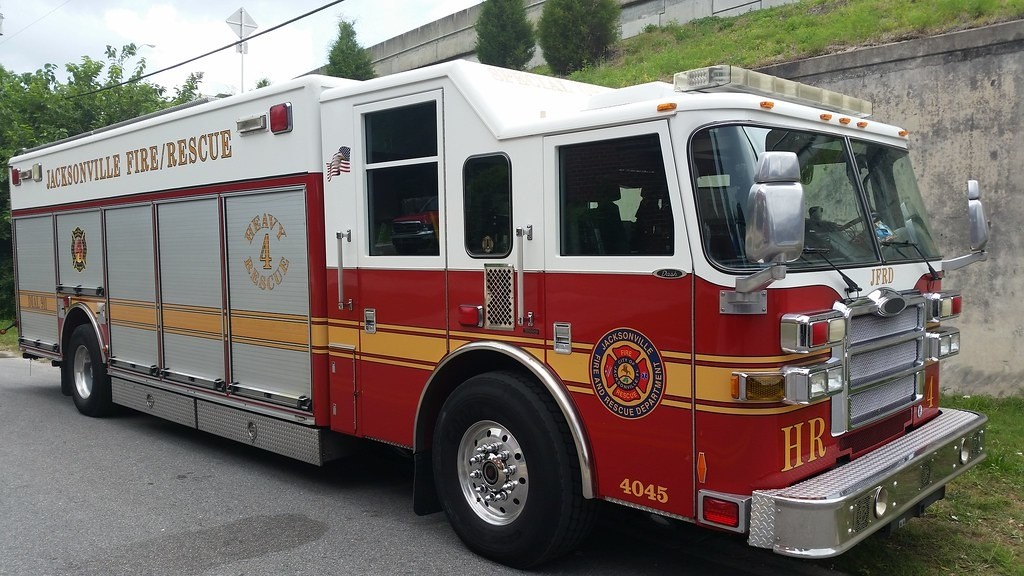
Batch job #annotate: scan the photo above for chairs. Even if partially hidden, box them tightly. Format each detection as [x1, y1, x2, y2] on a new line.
[580, 175, 675, 258]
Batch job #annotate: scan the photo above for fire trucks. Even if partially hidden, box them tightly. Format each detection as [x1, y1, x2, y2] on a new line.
[7, 56, 991, 565]
